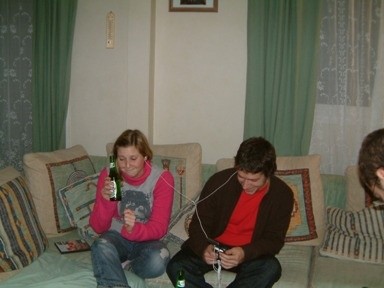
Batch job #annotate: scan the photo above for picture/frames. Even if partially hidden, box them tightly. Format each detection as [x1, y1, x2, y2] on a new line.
[169, 0, 218, 12]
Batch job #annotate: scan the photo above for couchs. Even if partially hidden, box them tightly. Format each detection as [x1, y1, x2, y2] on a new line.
[0, 155, 384, 288]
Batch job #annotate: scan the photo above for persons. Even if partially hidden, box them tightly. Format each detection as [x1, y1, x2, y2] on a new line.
[90, 129, 174, 288]
[358, 128, 384, 208]
[166, 136, 294, 288]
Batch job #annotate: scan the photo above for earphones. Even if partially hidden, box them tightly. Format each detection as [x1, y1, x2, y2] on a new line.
[144, 155, 148, 160]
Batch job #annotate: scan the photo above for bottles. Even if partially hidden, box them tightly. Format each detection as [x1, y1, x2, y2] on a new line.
[107, 152, 122, 201]
[175, 270, 186, 288]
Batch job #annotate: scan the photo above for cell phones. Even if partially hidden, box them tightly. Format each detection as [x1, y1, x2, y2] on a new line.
[212, 245, 230, 253]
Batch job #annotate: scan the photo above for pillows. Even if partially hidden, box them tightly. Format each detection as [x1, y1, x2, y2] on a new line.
[106, 143, 203, 241]
[59, 174, 100, 247]
[23, 145, 97, 239]
[216, 154, 326, 246]
[319, 205, 384, 264]
[0, 166, 49, 281]
[344, 165, 371, 209]
